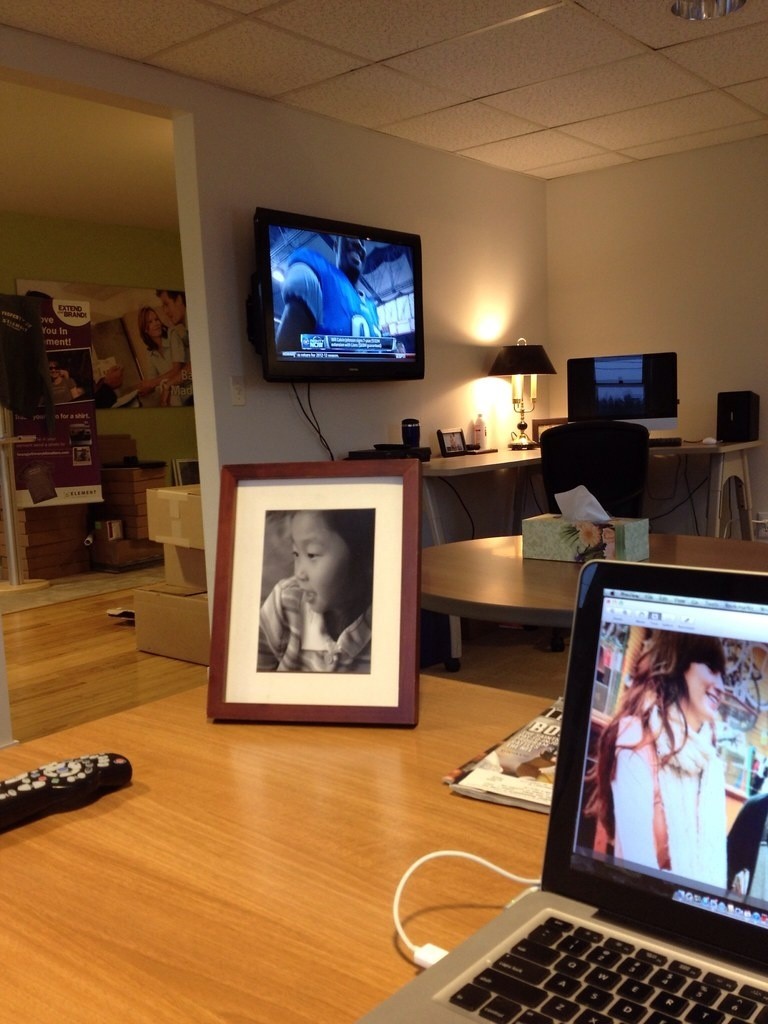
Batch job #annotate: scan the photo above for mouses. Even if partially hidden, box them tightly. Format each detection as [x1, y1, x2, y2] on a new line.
[702, 438, 718, 445]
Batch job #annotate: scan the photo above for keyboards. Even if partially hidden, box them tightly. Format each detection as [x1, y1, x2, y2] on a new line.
[648, 437, 682, 447]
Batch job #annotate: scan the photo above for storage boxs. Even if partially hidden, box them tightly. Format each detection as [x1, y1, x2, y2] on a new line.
[0, 434, 210, 666]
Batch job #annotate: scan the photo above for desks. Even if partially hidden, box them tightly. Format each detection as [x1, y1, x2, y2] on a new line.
[334, 437, 761, 672]
[411, 533, 768, 626]
[0, 677, 558, 1024]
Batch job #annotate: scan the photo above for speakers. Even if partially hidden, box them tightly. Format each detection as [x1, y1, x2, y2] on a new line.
[716, 390, 759, 442]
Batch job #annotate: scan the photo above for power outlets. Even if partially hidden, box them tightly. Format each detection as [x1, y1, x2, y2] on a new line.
[230, 375, 247, 407]
[757, 511, 768, 539]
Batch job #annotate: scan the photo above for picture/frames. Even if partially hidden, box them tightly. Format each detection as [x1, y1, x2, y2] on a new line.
[532, 418, 567, 443]
[208, 453, 421, 729]
[437, 427, 467, 457]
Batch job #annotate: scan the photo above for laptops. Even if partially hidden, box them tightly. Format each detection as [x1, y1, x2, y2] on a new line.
[353, 560, 768, 1024]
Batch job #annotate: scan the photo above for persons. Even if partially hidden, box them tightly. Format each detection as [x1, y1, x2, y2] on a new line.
[275, 235, 407, 357]
[583, 630, 728, 889]
[93, 365, 124, 409]
[256, 510, 375, 674]
[136, 290, 194, 407]
[48, 360, 77, 404]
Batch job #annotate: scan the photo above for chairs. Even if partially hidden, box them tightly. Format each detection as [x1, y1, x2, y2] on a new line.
[543, 421, 652, 518]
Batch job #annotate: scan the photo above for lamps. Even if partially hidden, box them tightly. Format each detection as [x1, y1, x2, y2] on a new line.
[484, 336, 556, 451]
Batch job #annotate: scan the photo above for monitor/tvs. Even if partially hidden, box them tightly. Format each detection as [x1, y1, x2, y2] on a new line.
[566, 352, 679, 429]
[246, 207, 425, 383]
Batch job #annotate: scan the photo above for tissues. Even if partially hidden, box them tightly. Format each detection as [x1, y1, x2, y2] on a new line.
[521, 486, 648, 564]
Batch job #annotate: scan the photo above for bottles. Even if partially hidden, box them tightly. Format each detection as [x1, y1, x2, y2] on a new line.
[474, 413, 487, 449]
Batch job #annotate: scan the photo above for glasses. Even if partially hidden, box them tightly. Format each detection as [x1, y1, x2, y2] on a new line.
[48, 366, 61, 370]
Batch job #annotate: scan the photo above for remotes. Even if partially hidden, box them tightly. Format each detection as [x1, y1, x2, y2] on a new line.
[0, 752, 132, 834]
